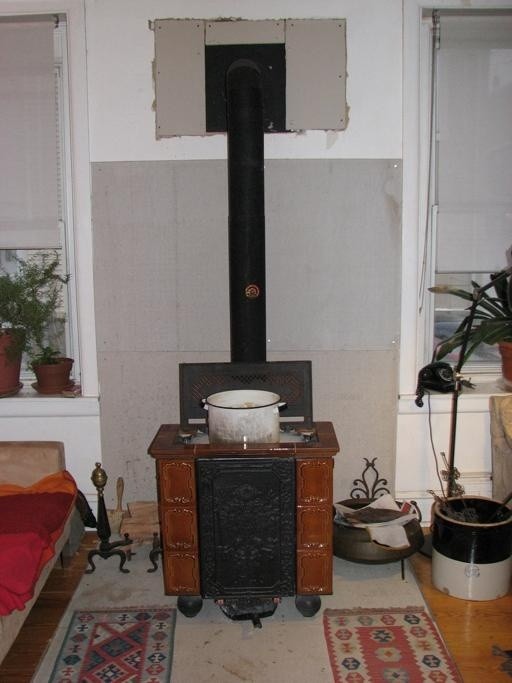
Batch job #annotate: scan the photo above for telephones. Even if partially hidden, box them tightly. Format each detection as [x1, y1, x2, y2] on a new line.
[418, 362, 456, 393]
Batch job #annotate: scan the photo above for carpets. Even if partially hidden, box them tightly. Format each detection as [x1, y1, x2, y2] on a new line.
[28, 532, 465, 683]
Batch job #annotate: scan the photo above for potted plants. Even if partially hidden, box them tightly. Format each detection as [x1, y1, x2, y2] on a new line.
[426, 270, 512, 392]
[0, 247, 79, 398]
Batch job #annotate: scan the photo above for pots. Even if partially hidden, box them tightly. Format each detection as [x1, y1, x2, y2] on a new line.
[199, 389, 285, 445]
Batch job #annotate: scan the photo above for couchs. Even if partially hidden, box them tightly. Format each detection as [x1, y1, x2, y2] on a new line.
[0, 439, 78, 668]
